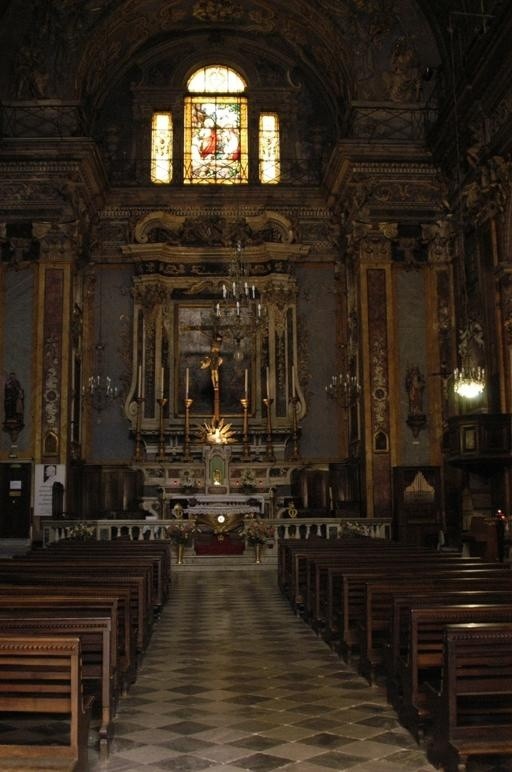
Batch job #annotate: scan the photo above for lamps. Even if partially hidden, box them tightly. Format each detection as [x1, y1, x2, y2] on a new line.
[452, 321, 487, 400]
[324, 372, 361, 413]
[81, 375, 118, 415]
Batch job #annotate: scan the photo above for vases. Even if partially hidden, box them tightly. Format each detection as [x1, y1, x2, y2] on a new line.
[253, 542, 263, 563]
[176, 543, 184, 565]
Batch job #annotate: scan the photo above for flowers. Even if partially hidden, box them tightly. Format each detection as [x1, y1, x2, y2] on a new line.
[165, 521, 203, 544]
[246, 520, 276, 547]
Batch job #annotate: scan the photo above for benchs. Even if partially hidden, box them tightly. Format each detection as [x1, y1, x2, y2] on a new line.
[278, 538, 512, 772]
[2, 541, 170, 770]
